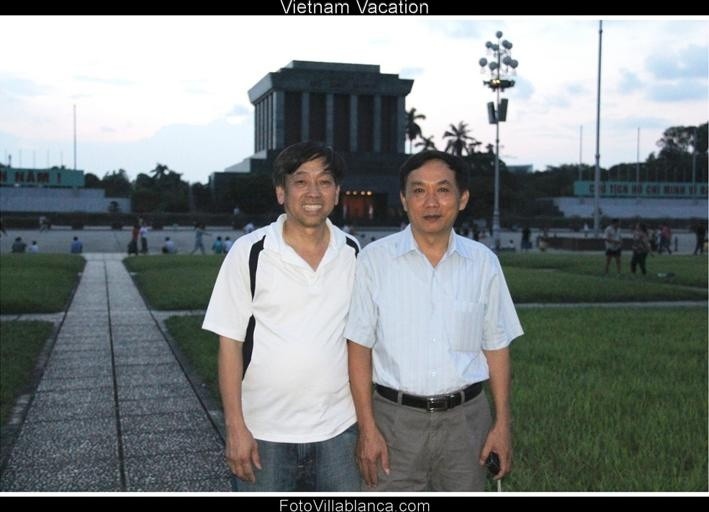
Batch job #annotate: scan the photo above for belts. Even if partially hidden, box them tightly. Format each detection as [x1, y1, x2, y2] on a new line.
[376, 378, 488, 413]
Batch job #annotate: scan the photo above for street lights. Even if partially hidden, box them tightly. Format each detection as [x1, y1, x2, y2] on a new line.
[476, 29, 521, 254]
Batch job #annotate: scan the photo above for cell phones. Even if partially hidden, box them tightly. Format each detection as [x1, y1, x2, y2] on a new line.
[486, 451, 501, 474]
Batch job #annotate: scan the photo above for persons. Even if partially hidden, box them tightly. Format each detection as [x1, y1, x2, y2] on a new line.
[342, 149, 525, 491]
[12, 236, 26, 253]
[343, 216, 407, 242]
[127, 204, 255, 255]
[202, 139, 362, 492]
[605, 218, 709, 275]
[39, 214, 51, 234]
[573, 216, 590, 237]
[459, 215, 550, 252]
[70, 236, 82, 254]
[29, 241, 39, 253]
